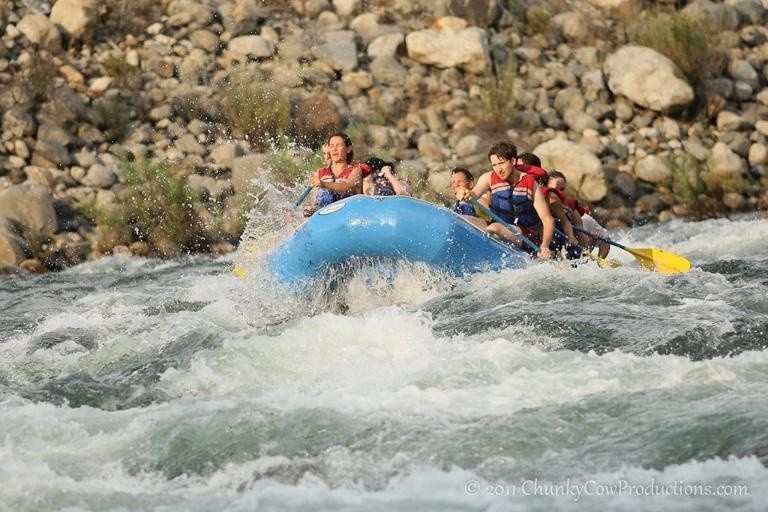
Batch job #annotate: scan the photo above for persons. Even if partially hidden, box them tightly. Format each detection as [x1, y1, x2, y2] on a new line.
[307, 134, 612, 265]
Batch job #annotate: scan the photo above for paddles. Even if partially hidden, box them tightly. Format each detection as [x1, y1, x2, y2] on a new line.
[573, 228, 691, 273]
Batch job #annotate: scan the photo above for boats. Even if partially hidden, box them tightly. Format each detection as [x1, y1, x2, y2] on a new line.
[256, 195, 613, 292]
